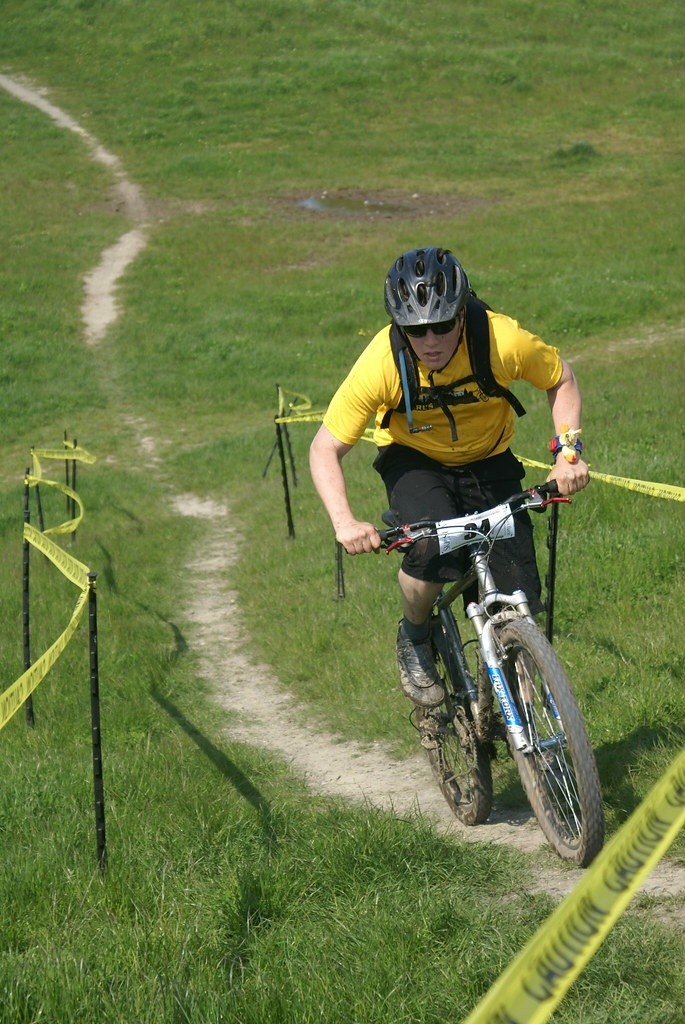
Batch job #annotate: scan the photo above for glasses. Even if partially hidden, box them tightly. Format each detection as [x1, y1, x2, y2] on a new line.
[403, 319, 456, 337]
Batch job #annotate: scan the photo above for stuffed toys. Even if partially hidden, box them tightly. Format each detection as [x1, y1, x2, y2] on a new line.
[559, 422, 582, 463]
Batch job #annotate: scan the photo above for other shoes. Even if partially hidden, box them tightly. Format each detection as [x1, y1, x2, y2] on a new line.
[395, 616, 447, 709]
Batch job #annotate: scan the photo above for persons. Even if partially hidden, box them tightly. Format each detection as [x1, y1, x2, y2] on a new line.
[308, 249, 589, 708]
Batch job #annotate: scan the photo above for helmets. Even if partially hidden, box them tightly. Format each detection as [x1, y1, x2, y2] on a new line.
[382, 247, 471, 326]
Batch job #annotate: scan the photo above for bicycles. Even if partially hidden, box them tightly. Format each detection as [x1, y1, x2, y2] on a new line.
[346, 479, 606, 868]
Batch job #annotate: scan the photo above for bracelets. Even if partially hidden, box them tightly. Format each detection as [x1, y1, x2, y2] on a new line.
[549, 436, 582, 454]
